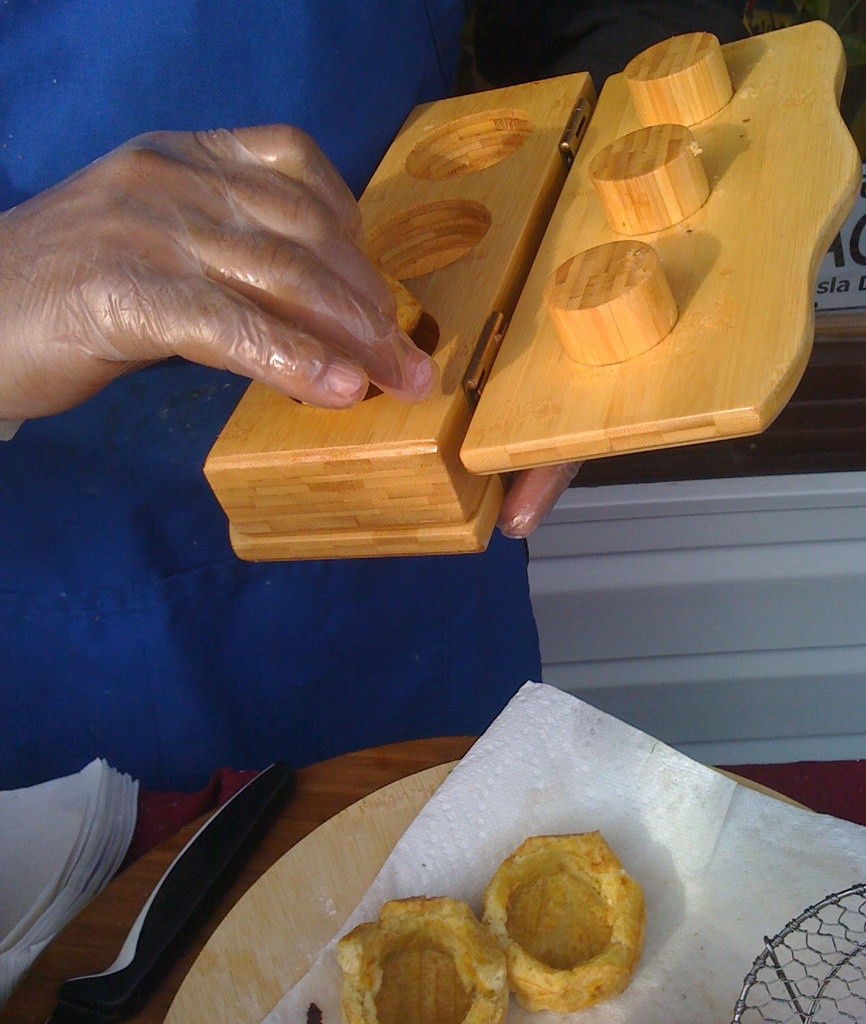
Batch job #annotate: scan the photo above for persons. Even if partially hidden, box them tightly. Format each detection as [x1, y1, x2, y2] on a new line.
[0, 0, 590, 792]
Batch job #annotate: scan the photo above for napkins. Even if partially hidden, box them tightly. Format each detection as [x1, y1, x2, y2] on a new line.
[0, 758, 140, 997]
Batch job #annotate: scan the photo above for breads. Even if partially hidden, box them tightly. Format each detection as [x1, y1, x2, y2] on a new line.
[335, 895, 511, 1024]
[482, 832, 647, 1013]
[379, 272, 423, 336]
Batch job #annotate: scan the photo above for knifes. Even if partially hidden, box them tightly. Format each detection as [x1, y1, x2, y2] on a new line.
[47, 754, 296, 1024]
